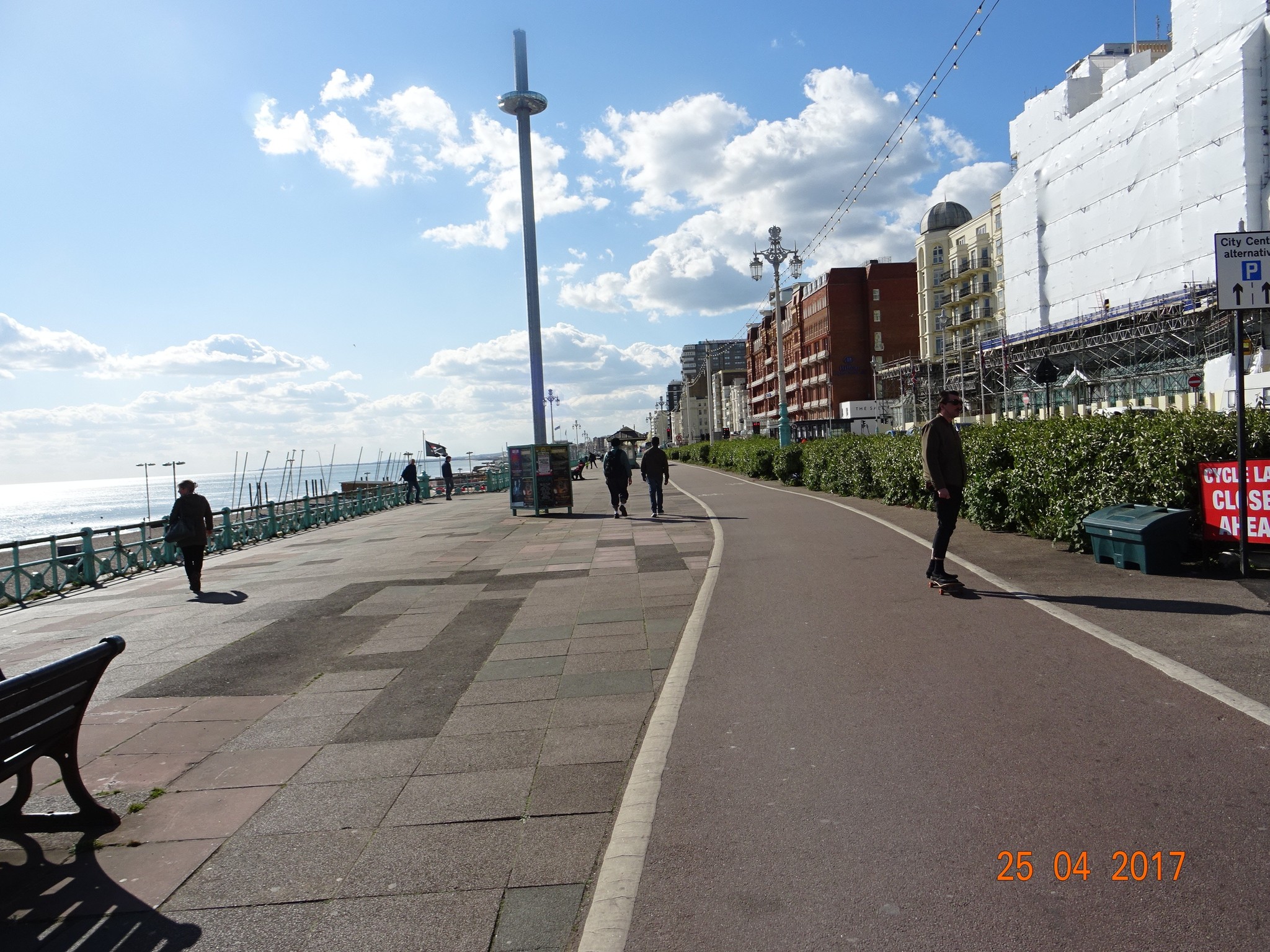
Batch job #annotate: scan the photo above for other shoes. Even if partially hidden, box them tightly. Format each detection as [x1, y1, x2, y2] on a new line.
[619, 505, 627, 517]
[657, 509, 664, 514]
[190, 583, 200, 592]
[651, 511, 657, 517]
[415, 500, 422, 503]
[931, 572, 959, 583]
[446, 496, 452, 500]
[406, 501, 412, 504]
[925, 570, 958, 579]
[614, 513, 619, 518]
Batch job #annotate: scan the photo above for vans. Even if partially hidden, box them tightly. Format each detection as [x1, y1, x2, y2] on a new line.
[1095, 407, 1163, 419]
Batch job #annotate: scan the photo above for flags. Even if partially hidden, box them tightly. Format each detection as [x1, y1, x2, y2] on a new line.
[425, 440, 448, 457]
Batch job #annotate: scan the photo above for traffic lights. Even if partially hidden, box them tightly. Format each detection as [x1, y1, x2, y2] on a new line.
[861, 420, 866, 428]
[667, 429, 670, 439]
[722, 428, 730, 439]
[881, 419, 884, 424]
[884, 418, 888, 425]
[752, 421, 761, 434]
[890, 420, 893, 426]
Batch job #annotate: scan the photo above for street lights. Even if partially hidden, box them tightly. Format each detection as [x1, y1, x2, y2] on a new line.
[655, 396, 669, 450]
[870, 355, 880, 436]
[135, 463, 156, 540]
[646, 413, 655, 441]
[162, 460, 186, 503]
[542, 388, 560, 443]
[747, 225, 802, 447]
[403, 452, 413, 465]
[572, 420, 582, 458]
[286, 458, 295, 512]
[938, 308, 949, 391]
[581, 430, 591, 455]
[466, 451, 473, 476]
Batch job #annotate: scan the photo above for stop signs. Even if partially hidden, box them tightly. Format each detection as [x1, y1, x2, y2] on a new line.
[1023, 392, 1029, 404]
[1188, 376, 1201, 387]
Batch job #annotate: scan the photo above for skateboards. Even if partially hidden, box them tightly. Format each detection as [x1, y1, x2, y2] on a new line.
[929, 576, 965, 595]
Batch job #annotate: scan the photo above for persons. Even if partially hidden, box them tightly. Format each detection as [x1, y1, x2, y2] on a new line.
[571, 459, 584, 481]
[442, 456, 454, 501]
[921, 390, 968, 583]
[599, 454, 604, 461]
[604, 438, 632, 519]
[402, 459, 423, 504]
[585, 452, 590, 469]
[587, 452, 598, 469]
[641, 436, 669, 517]
[168, 479, 214, 594]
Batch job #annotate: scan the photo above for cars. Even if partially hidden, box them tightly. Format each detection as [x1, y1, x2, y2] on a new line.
[885, 427, 922, 438]
[954, 422, 974, 435]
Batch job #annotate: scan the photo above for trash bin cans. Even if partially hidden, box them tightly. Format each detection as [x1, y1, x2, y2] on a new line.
[1082, 503, 1196, 575]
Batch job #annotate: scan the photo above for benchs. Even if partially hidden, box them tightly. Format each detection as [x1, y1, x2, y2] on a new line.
[572, 464, 585, 479]
[0, 635, 126, 829]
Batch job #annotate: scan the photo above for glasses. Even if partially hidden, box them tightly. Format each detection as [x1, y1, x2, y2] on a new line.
[948, 399, 962, 406]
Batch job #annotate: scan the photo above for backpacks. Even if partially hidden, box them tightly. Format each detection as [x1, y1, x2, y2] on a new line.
[603, 449, 624, 478]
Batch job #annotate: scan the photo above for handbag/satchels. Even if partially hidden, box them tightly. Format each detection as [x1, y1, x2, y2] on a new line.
[164, 519, 191, 543]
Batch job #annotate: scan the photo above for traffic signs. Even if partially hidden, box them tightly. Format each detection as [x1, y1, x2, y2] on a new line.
[1214, 230, 1270, 311]
